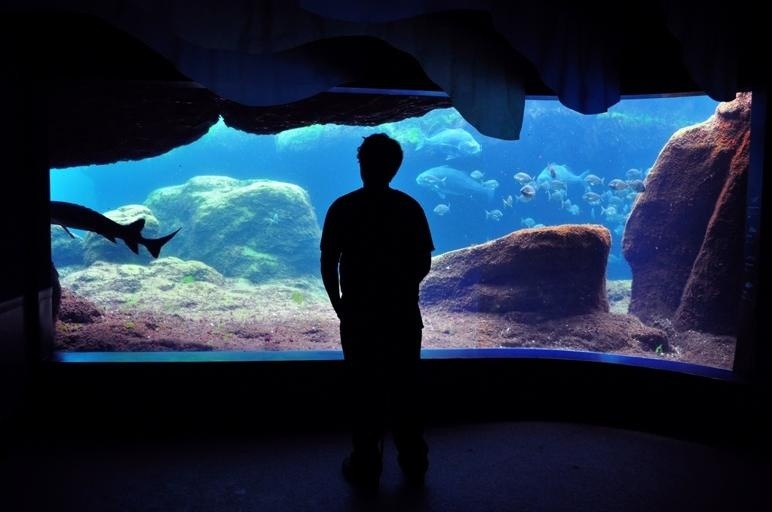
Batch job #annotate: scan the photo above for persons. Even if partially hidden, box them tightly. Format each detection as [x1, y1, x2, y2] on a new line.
[318, 131, 436, 504]
